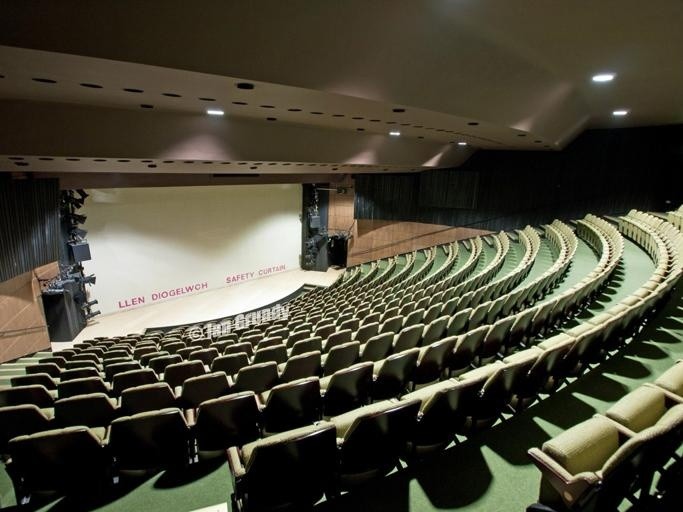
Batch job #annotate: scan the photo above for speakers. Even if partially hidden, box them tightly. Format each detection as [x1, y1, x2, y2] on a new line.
[310, 216, 321, 229]
[67, 240, 91, 263]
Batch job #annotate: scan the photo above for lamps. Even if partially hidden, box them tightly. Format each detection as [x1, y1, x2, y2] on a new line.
[60, 192, 84, 209]
